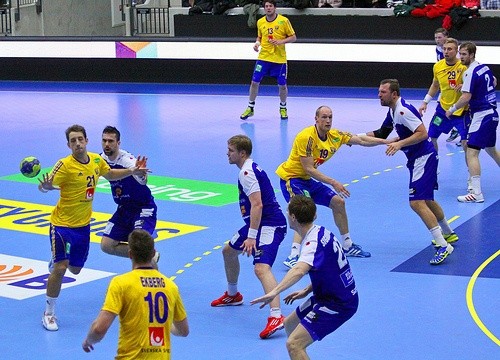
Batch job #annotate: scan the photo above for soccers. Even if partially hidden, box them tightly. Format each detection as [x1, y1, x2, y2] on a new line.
[19, 156, 41, 178]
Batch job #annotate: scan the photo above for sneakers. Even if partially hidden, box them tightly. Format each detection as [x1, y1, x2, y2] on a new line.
[446, 129, 460, 142]
[456, 141, 461, 146]
[430, 240, 454, 265]
[283, 254, 300, 269]
[240, 107, 254, 120]
[42, 311, 58, 331]
[342, 241, 371, 257]
[210, 290, 244, 307]
[279, 106, 288, 120]
[431, 230, 459, 245]
[48, 259, 54, 273]
[457, 189, 484, 203]
[259, 314, 287, 339]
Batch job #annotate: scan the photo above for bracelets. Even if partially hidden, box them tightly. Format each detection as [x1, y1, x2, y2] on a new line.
[450, 104, 457, 113]
[424, 95, 433, 104]
[42, 188, 48, 191]
[357, 133, 367, 135]
[256, 41, 260, 45]
[248, 228, 258, 239]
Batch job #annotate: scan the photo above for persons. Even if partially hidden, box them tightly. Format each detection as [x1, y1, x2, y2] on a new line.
[82, 229, 189, 360]
[346, 79, 459, 263]
[182, 0, 500, 10]
[275, 105, 400, 268]
[211, 135, 287, 338]
[435, 28, 461, 146]
[240, 0, 296, 119]
[251, 197, 359, 360]
[418, 38, 473, 191]
[446, 42, 500, 202]
[37, 124, 153, 331]
[100, 125, 160, 270]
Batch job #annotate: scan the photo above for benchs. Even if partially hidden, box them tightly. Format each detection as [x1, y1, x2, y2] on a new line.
[174, 0, 500, 45]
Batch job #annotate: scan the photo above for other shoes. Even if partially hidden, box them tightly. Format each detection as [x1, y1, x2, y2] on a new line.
[153, 251, 160, 263]
